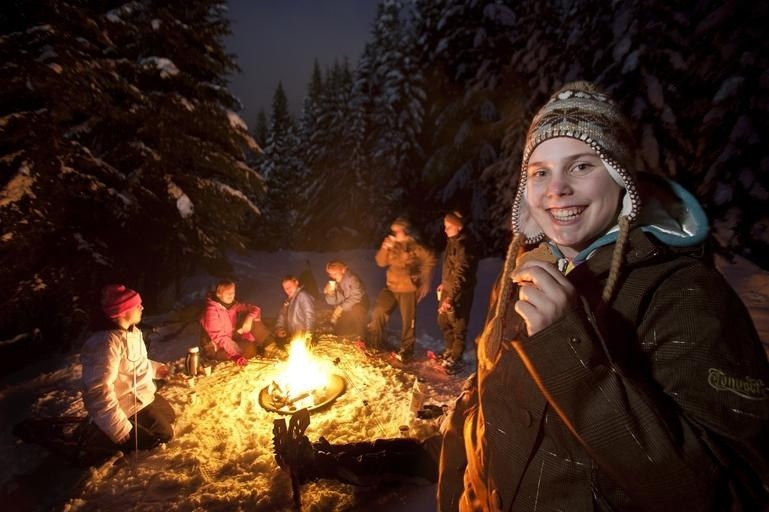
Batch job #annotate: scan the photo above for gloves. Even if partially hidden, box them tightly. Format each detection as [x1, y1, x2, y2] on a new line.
[441, 297, 454, 313]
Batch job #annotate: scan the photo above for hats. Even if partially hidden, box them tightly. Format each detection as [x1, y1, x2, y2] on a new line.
[512, 80, 641, 245]
[104, 285, 142, 319]
[445, 210, 464, 227]
[391, 217, 411, 229]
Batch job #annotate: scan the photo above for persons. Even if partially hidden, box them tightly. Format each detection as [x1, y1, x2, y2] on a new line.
[434, 77, 769, 509]
[436, 208, 480, 371]
[274, 272, 320, 349]
[70, 282, 179, 454]
[320, 261, 372, 341]
[371, 218, 438, 364]
[198, 280, 288, 369]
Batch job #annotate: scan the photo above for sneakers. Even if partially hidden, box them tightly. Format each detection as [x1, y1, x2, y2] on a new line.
[264, 342, 289, 361]
[436, 352, 457, 369]
[397, 349, 413, 362]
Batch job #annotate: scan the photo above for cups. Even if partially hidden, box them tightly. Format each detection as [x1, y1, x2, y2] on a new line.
[185, 375, 194, 387]
[387, 235, 396, 248]
[186, 389, 196, 406]
[328, 280, 336, 291]
[437, 291, 442, 302]
[200, 364, 212, 376]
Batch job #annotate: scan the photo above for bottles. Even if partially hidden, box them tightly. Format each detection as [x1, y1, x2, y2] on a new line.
[186, 345, 199, 375]
[408, 377, 427, 412]
[398, 424, 411, 438]
